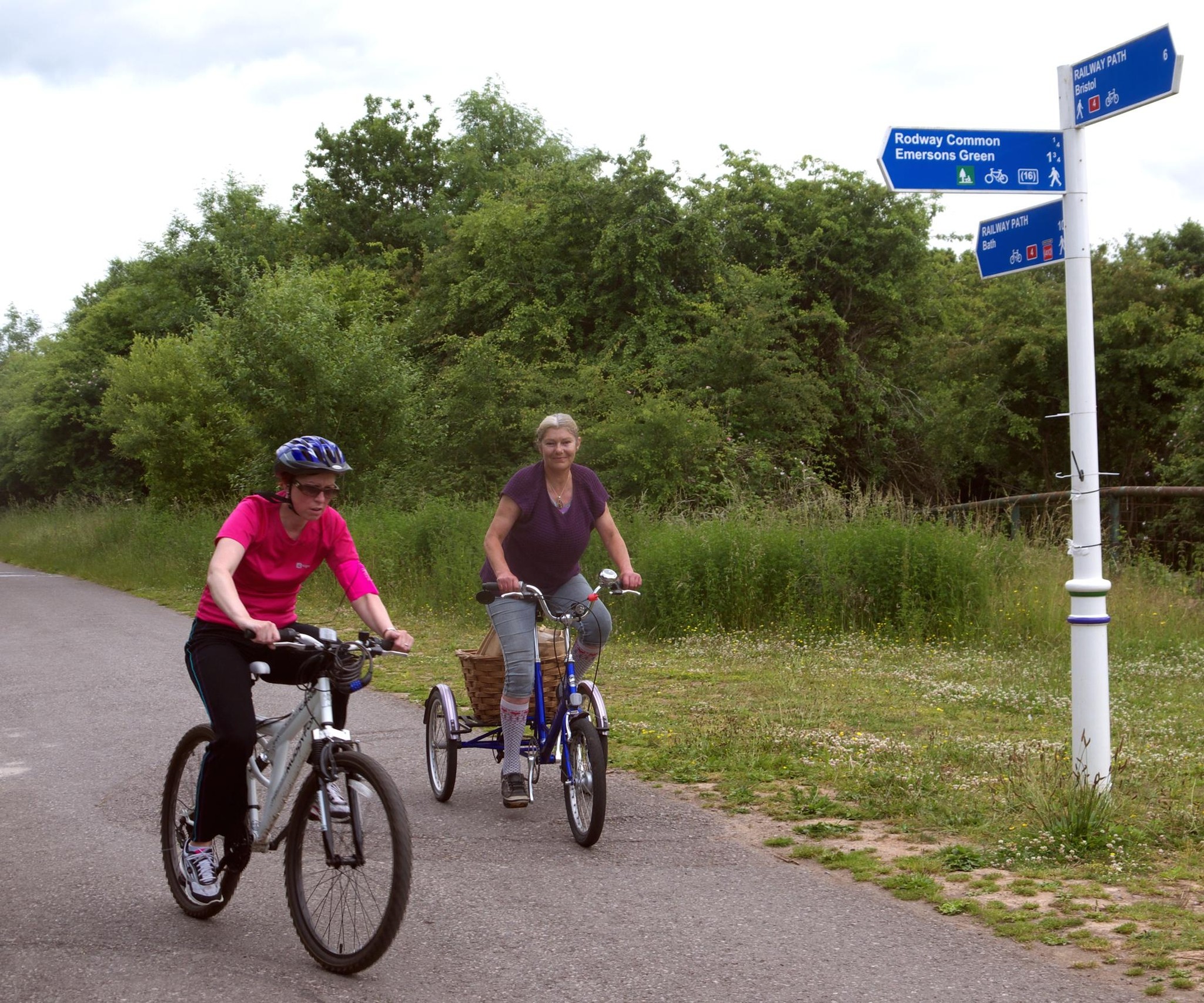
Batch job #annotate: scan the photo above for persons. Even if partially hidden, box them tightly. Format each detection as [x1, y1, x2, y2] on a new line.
[185, 436, 413, 906]
[479, 412, 643, 808]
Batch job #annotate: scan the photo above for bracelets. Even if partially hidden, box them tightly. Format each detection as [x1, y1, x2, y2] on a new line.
[382, 627, 397, 639]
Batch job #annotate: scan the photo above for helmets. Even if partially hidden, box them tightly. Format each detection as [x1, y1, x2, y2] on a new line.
[275, 435, 352, 473]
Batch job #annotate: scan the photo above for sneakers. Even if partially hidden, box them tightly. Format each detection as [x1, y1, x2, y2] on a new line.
[178, 837, 224, 907]
[305, 780, 351, 823]
[500, 768, 530, 804]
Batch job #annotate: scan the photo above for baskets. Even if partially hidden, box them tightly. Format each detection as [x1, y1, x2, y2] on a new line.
[454, 649, 567, 723]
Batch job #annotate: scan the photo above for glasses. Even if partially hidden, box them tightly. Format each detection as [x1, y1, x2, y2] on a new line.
[289, 478, 340, 499]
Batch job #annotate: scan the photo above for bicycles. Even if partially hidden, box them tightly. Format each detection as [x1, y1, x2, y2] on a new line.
[160, 633, 418, 980]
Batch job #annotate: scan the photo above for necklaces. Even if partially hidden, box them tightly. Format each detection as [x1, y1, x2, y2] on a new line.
[547, 470, 570, 509]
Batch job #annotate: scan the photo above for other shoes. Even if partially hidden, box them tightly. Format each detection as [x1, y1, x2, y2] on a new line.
[556, 683, 567, 702]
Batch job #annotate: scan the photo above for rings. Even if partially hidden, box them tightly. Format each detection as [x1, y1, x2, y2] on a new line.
[404, 643, 409, 646]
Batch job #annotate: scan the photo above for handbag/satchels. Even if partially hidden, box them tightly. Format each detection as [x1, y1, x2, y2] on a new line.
[476, 619, 567, 658]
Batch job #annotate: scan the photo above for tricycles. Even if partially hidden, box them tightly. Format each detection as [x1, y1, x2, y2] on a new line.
[413, 566, 615, 855]
